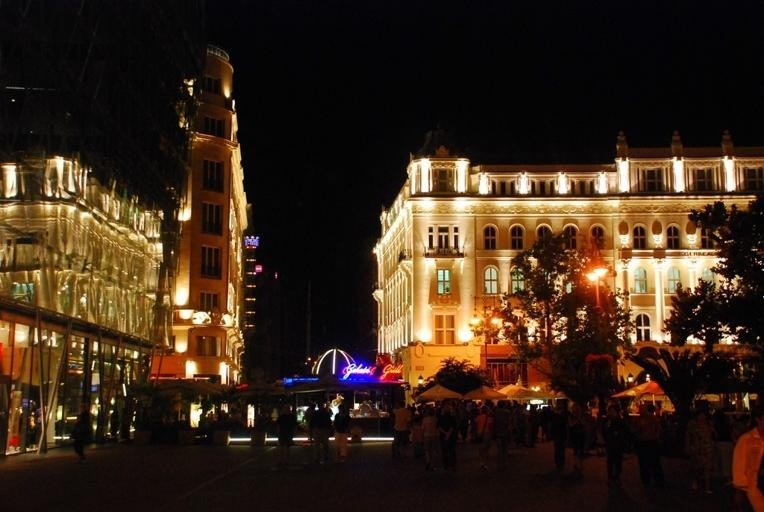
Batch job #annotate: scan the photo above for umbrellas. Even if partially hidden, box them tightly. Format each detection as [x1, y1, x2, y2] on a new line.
[611, 381, 666, 415]
[461, 386, 505, 401]
[414, 384, 464, 401]
[494, 382, 566, 400]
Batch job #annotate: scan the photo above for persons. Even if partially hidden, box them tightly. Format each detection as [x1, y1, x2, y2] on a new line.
[68, 404, 90, 455]
[121, 407, 130, 440]
[275, 404, 296, 465]
[199, 407, 240, 421]
[301, 399, 387, 438]
[309, 400, 333, 465]
[538, 399, 719, 495]
[332, 404, 353, 464]
[393, 399, 538, 471]
[710, 401, 763, 511]
[108, 407, 119, 437]
